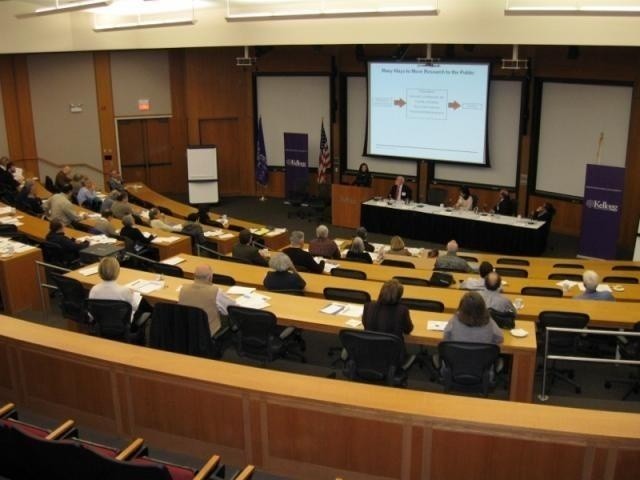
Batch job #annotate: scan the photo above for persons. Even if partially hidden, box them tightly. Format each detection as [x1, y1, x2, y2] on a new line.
[230, 226, 519, 325]
[179, 262, 237, 337]
[351, 163, 372, 188]
[528, 198, 556, 222]
[361, 277, 415, 364]
[432, 291, 505, 374]
[387, 176, 412, 201]
[572, 269, 616, 301]
[454, 186, 474, 212]
[1, 161, 228, 325]
[491, 190, 513, 216]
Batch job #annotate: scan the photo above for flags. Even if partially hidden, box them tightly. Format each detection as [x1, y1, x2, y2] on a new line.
[316, 115, 331, 198]
[254, 115, 270, 187]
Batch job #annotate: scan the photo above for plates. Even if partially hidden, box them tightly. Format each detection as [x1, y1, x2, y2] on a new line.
[511, 328, 528, 338]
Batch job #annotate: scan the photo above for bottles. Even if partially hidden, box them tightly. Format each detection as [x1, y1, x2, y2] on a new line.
[563, 280, 569, 296]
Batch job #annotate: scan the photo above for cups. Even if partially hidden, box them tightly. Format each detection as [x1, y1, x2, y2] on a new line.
[613, 284, 625, 291]
[514, 297, 525, 308]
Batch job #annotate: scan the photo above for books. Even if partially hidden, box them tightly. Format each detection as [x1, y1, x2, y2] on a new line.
[577, 282, 613, 293]
[426, 321, 448, 331]
